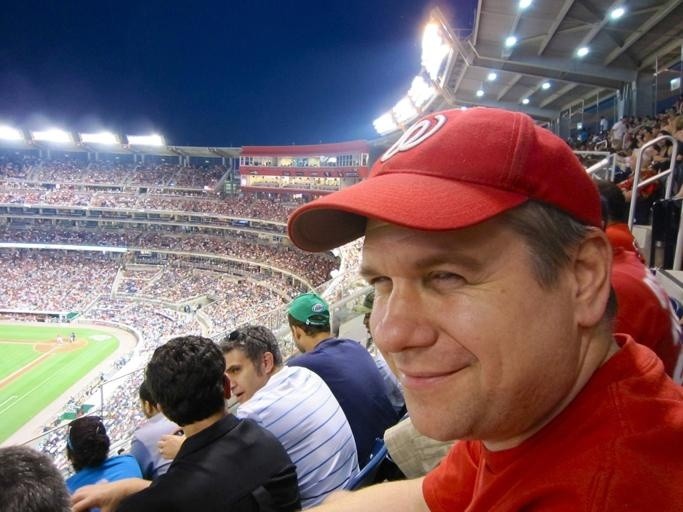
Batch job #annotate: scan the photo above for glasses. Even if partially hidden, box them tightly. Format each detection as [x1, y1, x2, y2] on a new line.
[227, 330, 274, 351]
[67, 415, 104, 452]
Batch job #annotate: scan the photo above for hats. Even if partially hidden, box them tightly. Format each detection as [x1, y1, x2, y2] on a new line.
[289, 293, 330, 326]
[355, 293, 378, 313]
[288, 109, 602, 254]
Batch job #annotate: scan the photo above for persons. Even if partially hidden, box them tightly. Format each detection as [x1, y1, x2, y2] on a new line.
[233, 179, 338, 227]
[600, 116, 608, 135]
[149, 223, 342, 358]
[296, 107, 681, 512]
[594, 181, 646, 267]
[0, 216, 148, 251]
[220, 324, 361, 511]
[660, 99, 682, 131]
[607, 115, 629, 152]
[287, 294, 398, 469]
[124, 378, 182, 478]
[71, 336, 302, 512]
[0, 250, 149, 336]
[0, 151, 234, 219]
[600, 197, 682, 390]
[629, 111, 659, 148]
[567, 129, 609, 157]
[66, 418, 144, 511]
[38, 425, 75, 479]
[617, 149, 658, 203]
[91, 372, 147, 456]
[654, 131, 683, 198]
[0, 447, 69, 512]
[362, 293, 409, 419]
